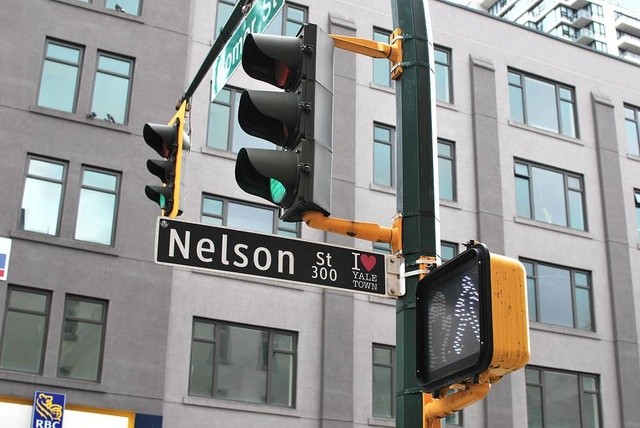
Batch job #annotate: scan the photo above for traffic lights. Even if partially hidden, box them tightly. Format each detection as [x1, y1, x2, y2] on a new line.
[142, 116, 179, 215]
[234, 22, 314, 253]
[415, 241, 491, 393]
